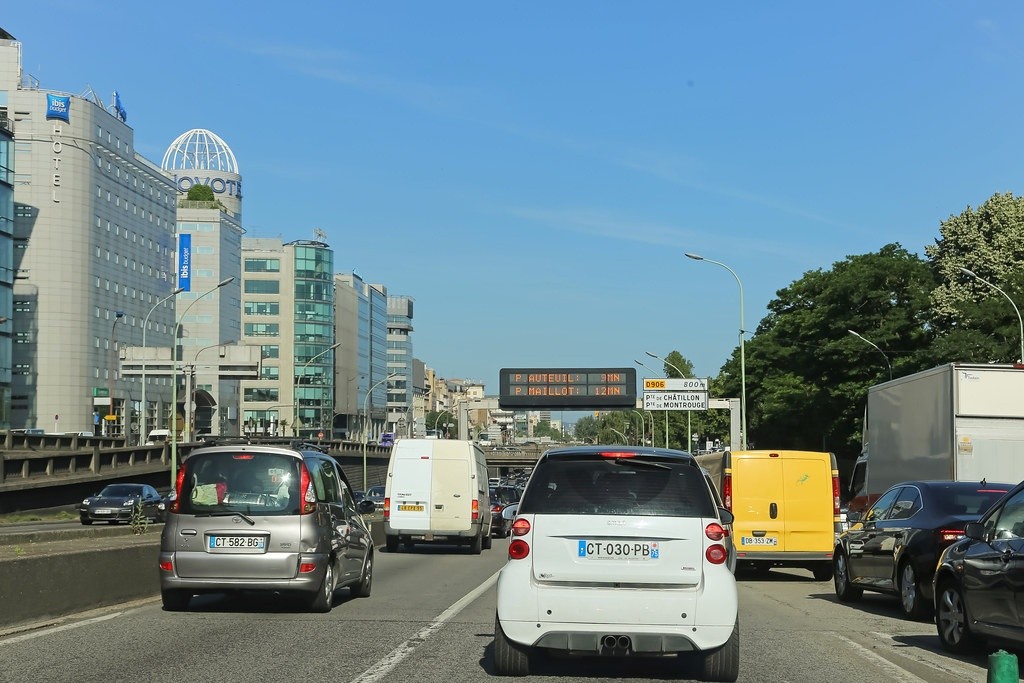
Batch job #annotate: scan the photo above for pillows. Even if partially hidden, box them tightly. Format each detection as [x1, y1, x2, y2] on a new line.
[191, 481, 228, 506]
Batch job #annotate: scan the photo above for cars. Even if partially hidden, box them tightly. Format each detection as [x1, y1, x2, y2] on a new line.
[489, 468, 531, 538]
[80, 483, 160, 525]
[832, 480, 1017, 620]
[932, 479, 1024, 655]
[143, 493, 169, 523]
[353, 486, 384, 508]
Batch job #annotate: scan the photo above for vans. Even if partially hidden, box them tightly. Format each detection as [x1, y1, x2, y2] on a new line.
[491, 445, 740, 683]
[382, 438, 494, 556]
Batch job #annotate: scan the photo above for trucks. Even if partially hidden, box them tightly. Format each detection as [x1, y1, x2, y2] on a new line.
[842, 361, 1024, 529]
[722, 448, 843, 583]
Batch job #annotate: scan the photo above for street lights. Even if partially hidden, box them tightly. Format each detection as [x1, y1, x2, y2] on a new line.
[405, 391, 432, 439]
[646, 350, 693, 453]
[434, 404, 458, 438]
[296, 341, 341, 438]
[363, 371, 400, 492]
[685, 252, 749, 452]
[958, 267, 1024, 364]
[611, 359, 670, 450]
[170, 275, 236, 492]
[140, 287, 187, 446]
[848, 329, 894, 381]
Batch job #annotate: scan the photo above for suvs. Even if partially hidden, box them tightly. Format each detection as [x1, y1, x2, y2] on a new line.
[159, 443, 377, 614]
